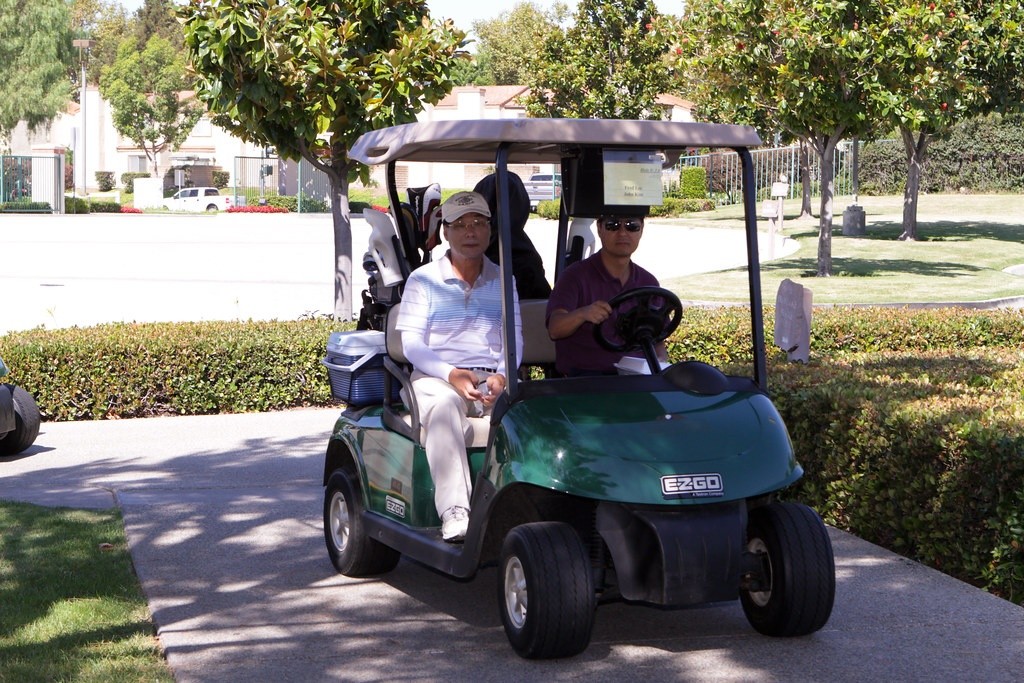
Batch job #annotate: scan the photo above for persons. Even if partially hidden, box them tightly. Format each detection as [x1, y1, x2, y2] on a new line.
[545, 212, 676, 383]
[395, 189, 525, 542]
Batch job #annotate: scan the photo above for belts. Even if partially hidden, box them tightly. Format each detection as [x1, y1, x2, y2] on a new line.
[456, 367, 496, 374]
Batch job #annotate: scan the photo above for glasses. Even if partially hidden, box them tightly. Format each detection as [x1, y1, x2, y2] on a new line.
[445, 219, 490, 230]
[601, 219, 642, 231]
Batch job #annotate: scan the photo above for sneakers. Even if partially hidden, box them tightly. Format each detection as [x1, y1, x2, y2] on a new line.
[441, 506, 469, 541]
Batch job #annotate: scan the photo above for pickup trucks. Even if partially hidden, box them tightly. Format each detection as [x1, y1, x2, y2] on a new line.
[523, 174, 562, 213]
[163, 186, 247, 213]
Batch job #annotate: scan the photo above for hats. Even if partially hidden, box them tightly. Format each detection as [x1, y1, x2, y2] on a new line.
[442, 191, 491, 222]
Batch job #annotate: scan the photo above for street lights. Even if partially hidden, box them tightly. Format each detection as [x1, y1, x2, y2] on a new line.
[72, 40, 89, 196]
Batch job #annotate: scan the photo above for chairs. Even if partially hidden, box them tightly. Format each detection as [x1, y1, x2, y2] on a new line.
[519, 300, 557, 364]
[383, 304, 493, 449]
